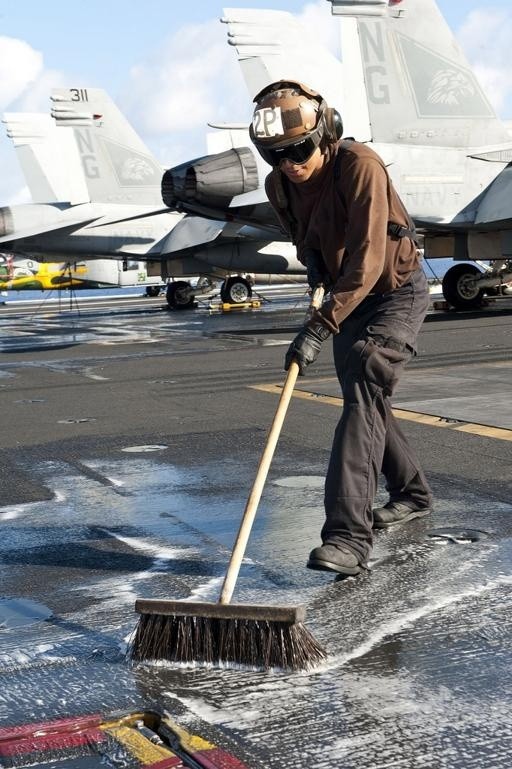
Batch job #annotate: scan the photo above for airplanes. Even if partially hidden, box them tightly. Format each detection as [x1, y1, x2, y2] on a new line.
[151, 1, 512, 314]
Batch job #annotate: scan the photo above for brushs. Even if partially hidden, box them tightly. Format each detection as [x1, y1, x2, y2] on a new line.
[126, 289, 331, 673]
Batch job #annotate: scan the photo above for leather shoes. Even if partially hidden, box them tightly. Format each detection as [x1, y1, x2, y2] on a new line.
[372, 498, 432, 528]
[305, 537, 367, 576]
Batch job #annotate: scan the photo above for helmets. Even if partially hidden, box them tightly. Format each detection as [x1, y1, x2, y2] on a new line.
[249, 78, 344, 149]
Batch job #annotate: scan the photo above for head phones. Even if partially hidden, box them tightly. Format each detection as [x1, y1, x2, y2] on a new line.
[318, 99, 344, 142]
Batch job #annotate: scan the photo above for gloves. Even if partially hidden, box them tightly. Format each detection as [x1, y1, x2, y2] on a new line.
[284, 314, 333, 376]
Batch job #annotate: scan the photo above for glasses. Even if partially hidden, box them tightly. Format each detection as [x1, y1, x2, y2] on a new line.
[248, 119, 329, 169]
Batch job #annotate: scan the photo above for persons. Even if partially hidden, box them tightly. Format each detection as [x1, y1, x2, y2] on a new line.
[247, 75, 438, 578]
